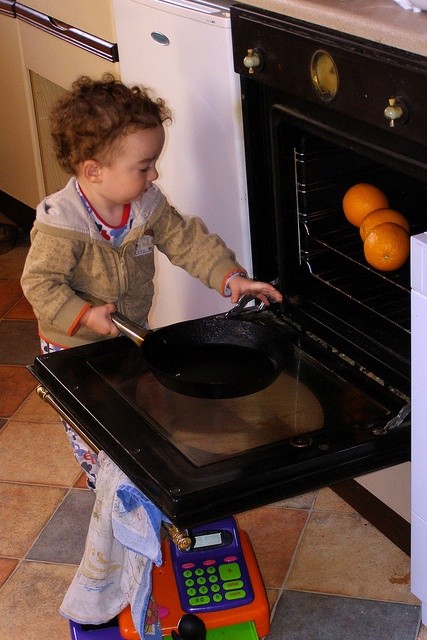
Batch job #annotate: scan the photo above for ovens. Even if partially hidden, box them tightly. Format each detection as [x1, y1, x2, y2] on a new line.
[23, 4, 426, 552]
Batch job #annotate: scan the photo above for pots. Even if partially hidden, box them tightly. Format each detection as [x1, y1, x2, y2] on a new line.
[111, 316, 284, 399]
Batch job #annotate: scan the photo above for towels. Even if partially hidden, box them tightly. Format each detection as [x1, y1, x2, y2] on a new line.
[58, 450, 174, 640]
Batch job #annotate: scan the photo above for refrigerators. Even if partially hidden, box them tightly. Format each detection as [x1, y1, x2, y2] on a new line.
[115, 1, 250, 324]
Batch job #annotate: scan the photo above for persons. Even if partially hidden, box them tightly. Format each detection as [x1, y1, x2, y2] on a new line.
[18, 72, 284, 490]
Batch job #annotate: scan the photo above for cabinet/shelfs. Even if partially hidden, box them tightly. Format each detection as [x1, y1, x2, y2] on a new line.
[0, 0, 75, 210]
[410, 230, 426, 628]
[14, 0, 121, 91]
